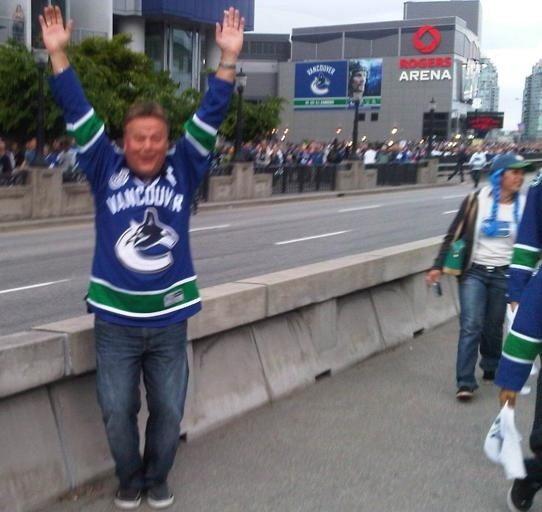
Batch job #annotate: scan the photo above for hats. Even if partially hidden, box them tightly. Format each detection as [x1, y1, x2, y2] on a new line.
[489, 153, 532, 174]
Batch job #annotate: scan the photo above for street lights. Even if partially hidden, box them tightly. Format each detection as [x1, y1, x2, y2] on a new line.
[351, 84, 363, 159]
[429, 98, 437, 157]
[234, 68, 248, 160]
[31, 35, 50, 167]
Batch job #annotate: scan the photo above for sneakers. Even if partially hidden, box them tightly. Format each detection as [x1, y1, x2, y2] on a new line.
[506, 458, 535, 512]
[114, 485, 146, 508]
[455, 386, 477, 400]
[145, 482, 174, 508]
[483, 369, 495, 385]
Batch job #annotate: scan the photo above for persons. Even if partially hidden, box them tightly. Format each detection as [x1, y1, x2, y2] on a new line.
[39, 3, 245, 510]
[348, 61, 368, 98]
[492, 263, 542, 511]
[211, 136, 541, 187]
[12, 4, 24, 41]
[1, 137, 80, 187]
[505, 171, 542, 310]
[425, 152, 542, 400]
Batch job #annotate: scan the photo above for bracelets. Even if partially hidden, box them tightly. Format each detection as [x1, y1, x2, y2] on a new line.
[218, 61, 235, 69]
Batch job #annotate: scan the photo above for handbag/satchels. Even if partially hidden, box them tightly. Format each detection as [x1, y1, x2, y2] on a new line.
[442, 238, 466, 277]
